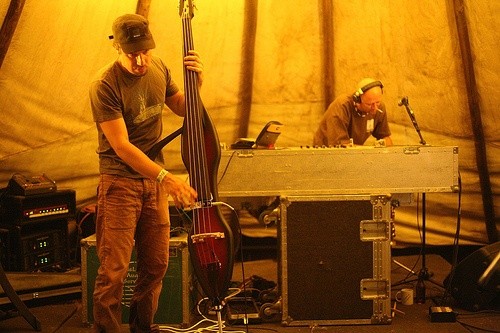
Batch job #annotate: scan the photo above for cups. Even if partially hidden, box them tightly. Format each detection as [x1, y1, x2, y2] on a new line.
[395, 289, 413, 305]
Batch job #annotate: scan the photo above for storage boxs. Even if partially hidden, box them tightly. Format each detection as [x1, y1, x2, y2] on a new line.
[80, 231, 197, 327]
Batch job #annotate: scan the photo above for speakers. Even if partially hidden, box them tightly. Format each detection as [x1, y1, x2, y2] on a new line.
[443, 241, 500, 312]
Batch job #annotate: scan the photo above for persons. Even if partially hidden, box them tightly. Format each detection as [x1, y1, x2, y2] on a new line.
[313, 77, 394, 146]
[88, 13, 204, 332]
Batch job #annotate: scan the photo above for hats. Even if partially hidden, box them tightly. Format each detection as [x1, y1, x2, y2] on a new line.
[108, 13, 156, 54]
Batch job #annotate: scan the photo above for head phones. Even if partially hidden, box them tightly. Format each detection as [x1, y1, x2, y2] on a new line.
[352, 80, 385, 104]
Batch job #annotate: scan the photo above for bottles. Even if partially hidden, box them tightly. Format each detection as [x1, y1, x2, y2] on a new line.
[415, 273, 425, 305]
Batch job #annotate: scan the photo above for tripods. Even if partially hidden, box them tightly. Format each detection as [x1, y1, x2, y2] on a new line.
[391, 101, 445, 289]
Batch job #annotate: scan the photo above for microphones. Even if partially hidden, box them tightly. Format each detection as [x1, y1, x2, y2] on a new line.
[398, 96, 408, 106]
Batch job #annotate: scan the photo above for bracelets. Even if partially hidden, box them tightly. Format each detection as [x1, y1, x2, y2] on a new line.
[156, 168, 168, 185]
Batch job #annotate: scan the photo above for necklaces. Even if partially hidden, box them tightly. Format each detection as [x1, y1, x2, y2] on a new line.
[353, 101, 370, 118]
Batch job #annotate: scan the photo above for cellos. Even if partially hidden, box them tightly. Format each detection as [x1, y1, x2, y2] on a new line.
[178, 0, 237, 333]
